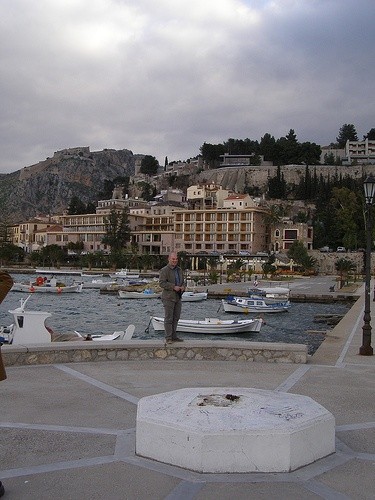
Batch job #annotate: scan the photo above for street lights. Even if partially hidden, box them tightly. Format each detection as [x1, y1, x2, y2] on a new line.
[358, 172, 375, 355]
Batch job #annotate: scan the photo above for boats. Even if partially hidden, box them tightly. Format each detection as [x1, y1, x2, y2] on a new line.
[149, 315, 259, 334]
[181, 292, 208, 302]
[11, 281, 82, 293]
[221, 287, 292, 312]
[81, 273, 162, 299]
[0, 309, 135, 341]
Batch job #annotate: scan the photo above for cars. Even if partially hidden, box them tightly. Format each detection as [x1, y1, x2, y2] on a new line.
[322, 245, 333, 252]
[337, 246, 347, 252]
[195, 249, 269, 257]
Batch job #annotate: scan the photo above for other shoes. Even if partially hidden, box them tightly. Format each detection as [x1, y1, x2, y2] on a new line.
[166, 335, 178, 343]
[173, 331, 184, 342]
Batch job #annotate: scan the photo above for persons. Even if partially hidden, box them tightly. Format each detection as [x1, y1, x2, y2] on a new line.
[159, 251, 185, 344]
[0, 268, 13, 499]
